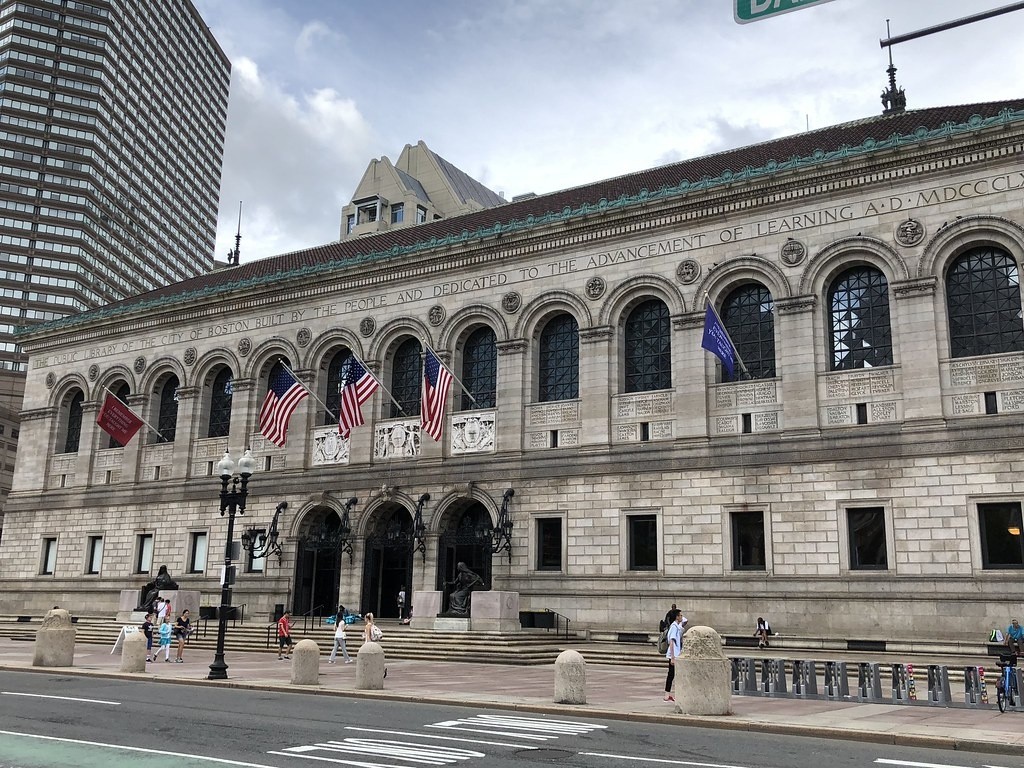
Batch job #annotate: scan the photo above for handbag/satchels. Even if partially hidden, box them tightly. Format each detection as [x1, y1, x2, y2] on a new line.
[173, 617, 180, 635]
[989, 630, 1003, 642]
[156, 611, 159, 618]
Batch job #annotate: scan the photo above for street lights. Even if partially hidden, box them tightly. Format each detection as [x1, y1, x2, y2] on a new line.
[204, 444, 257, 681]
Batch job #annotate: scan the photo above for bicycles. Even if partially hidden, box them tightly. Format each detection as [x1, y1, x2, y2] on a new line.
[995, 652, 1017, 714]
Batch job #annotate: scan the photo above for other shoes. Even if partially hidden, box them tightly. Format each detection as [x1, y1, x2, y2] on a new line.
[329, 661, 336, 664]
[345, 660, 353, 664]
[765, 641, 769, 646]
[146, 659, 151, 663]
[284, 656, 290, 659]
[153, 653, 157, 660]
[761, 643, 764, 648]
[164, 659, 171, 663]
[174, 658, 183, 663]
[383, 668, 388, 678]
[663, 696, 675, 702]
[278, 657, 283, 660]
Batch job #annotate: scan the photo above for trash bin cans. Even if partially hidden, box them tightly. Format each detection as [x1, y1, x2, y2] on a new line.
[274, 604, 284, 621]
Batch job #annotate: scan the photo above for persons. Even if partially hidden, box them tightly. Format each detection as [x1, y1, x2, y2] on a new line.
[140, 597, 193, 663]
[363, 613, 383, 644]
[133, 565, 179, 612]
[658, 603, 686, 700]
[1003, 619, 1024, 656]
[277, 610, 296, 658]
[329, 612, 353, 664]
[397, 586, 406, 619]
[753, 617, 772, 647]
[441, 562, 484, 613]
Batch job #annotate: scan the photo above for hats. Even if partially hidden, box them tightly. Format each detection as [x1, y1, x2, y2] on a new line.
[145, 614, 152, 619]
[284, 610, 291, 614]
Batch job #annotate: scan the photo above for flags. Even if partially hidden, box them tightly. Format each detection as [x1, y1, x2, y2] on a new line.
[335, 352, 380, 442]
[701, 303, 735, 378]
[257, 368, 309, 448]
[96, 391, 145, 447]
[419, 340, 450, 435]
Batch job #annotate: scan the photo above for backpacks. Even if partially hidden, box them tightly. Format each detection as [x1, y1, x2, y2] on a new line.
[367, 623, 382, 640]
[657, 624, 678, 654]
